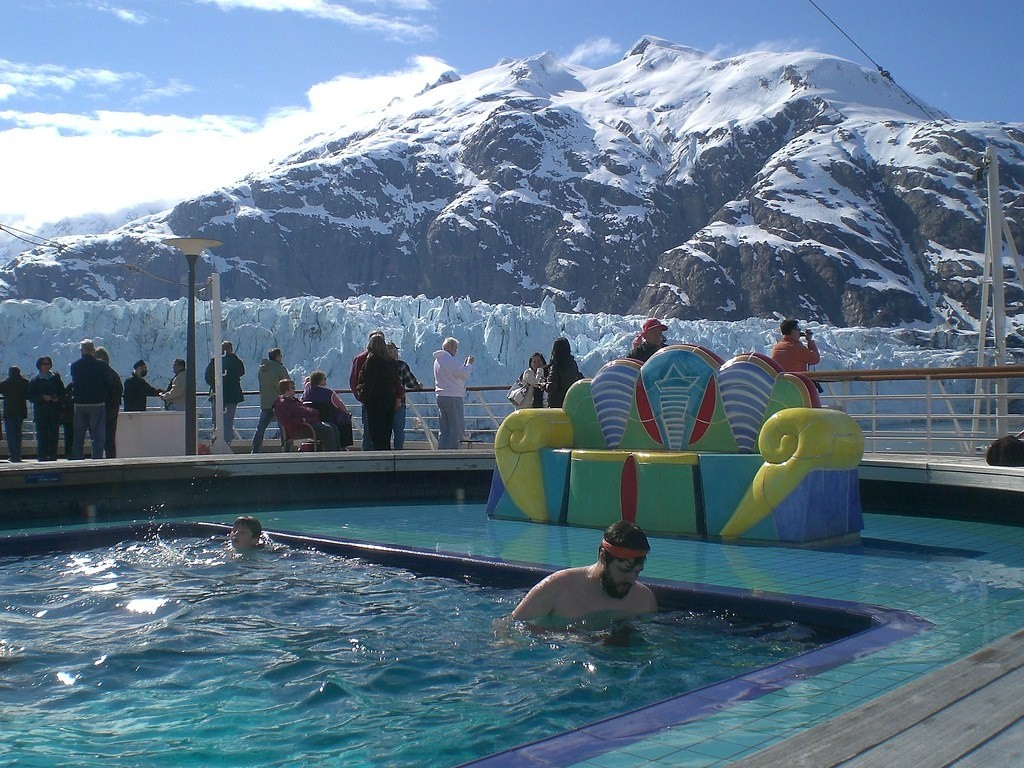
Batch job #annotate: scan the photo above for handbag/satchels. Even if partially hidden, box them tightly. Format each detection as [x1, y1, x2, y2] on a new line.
[506, 371, 530, 405]
[359, 354, 389, 409]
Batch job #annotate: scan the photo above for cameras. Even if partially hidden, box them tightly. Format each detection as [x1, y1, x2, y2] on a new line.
[799, 332, 807, 337]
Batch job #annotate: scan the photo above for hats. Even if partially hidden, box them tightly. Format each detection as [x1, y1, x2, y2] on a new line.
[386, 342, 400, 349]
[643, 318, 668, 333]
[133, 360, 145, 370]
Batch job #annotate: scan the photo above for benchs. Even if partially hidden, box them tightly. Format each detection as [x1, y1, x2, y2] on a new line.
[487, 343, 867, 547]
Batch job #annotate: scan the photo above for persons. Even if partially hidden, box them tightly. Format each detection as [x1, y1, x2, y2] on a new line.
[511, 521, 658, 627]
[772, 319, 821, 373]
[123, 360, 163, 411]
[205, 341, 245, 445]
[514, 353, 550, 410]
[230, 515, 262, 550]
[350, 330, 405, 450]
[300, 372, 354, 449]
[536, 337, 582, 409]
[93, 347, 123, 458]
[159, 358, 187, 411]
[385, 341, 424, 449]
[251, 348, 292, 452]
[2, 365, 30, 461]
[28, 356, 66, 461]
[69, 339, 114, 459]
[274, 378, 343, 452]
[432, 337, 475, 448]
[356, 335, 398, 450]
[627, 318, 672, 363]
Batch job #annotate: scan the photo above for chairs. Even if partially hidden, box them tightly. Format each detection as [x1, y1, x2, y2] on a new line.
[273, 409, 320, 453]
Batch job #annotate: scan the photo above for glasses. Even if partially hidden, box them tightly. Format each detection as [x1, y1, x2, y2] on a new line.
[796, 328, 801, 332]
[42, 362, 51, 366]
